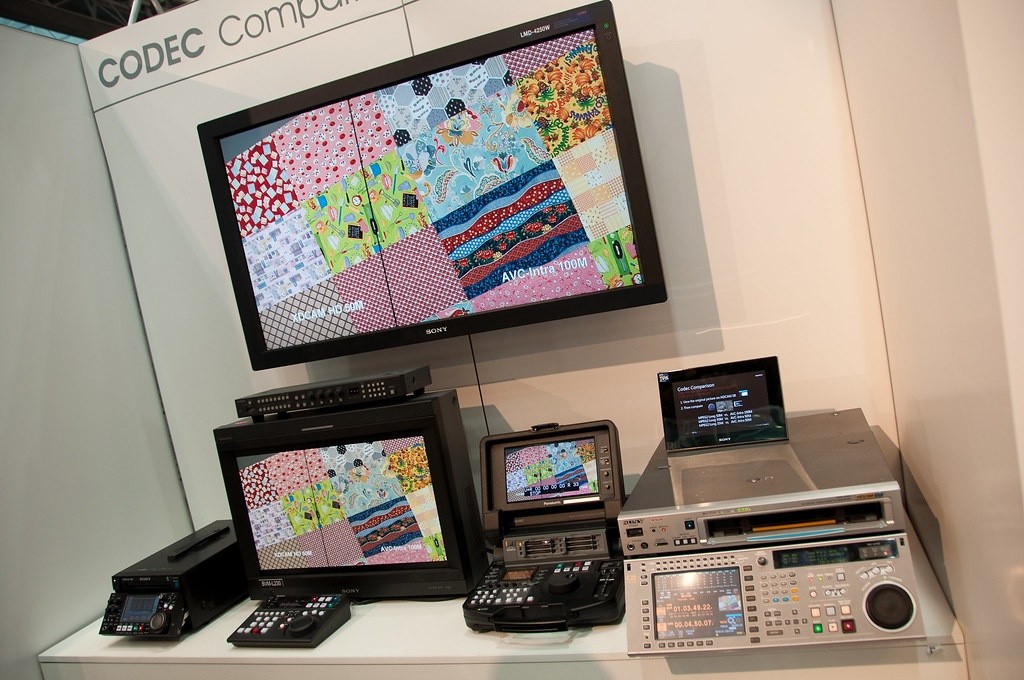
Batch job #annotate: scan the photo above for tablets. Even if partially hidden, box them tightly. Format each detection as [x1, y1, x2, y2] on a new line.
[657, 356, 789, 454]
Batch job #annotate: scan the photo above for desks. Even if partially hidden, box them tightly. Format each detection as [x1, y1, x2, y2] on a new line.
[34, 509, 968, 680]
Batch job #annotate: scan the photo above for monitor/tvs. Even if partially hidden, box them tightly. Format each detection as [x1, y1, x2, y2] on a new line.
[214, 389, 490, 604]
[504, 436, 599, 504]
[198, 0, 669, 371]
